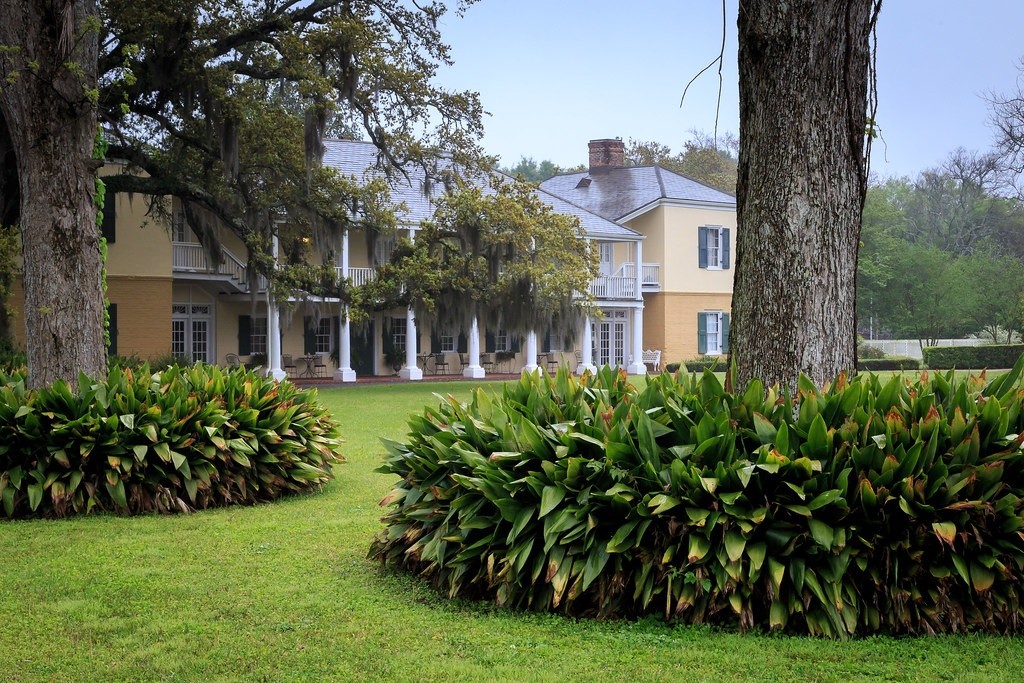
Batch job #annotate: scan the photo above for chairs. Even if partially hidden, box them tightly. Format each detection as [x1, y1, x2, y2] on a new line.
[282, 354, 297, 378]
[226, 353, 247, 369]
[434, 353, 450, 375]
[459, 353, 469, 374]
[482, 354, 494, 375]
[575, 350, 583, 368]
[313, 355, 327, 377]
[546, 352, 558, 374]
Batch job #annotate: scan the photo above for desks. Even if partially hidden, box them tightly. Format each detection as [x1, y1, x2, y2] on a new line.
[299, 357, 319, 378]
[417, 356, 435, 375]
[537, 354, 547, 366]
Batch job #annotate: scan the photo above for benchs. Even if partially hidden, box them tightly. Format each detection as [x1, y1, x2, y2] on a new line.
[628, 349, 661, 372]
